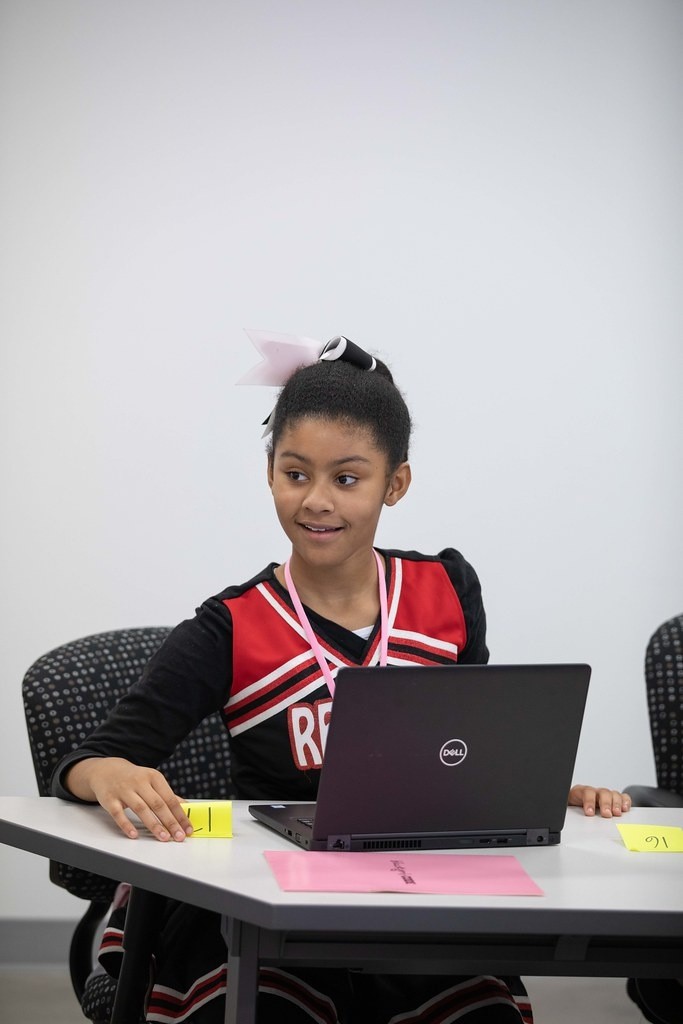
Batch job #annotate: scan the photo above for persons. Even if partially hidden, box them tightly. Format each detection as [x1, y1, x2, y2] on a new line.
[52, 336, 633, 1024]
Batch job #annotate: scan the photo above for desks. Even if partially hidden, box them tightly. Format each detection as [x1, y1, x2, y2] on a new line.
[0, 795, 683, 1024]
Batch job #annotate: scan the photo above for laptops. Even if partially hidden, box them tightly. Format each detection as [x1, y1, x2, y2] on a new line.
[248, 662, 592, 852]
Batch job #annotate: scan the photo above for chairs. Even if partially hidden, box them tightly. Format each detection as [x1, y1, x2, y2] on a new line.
[622, 615, 683, 1024]
[22, 627, 232, 1024]
